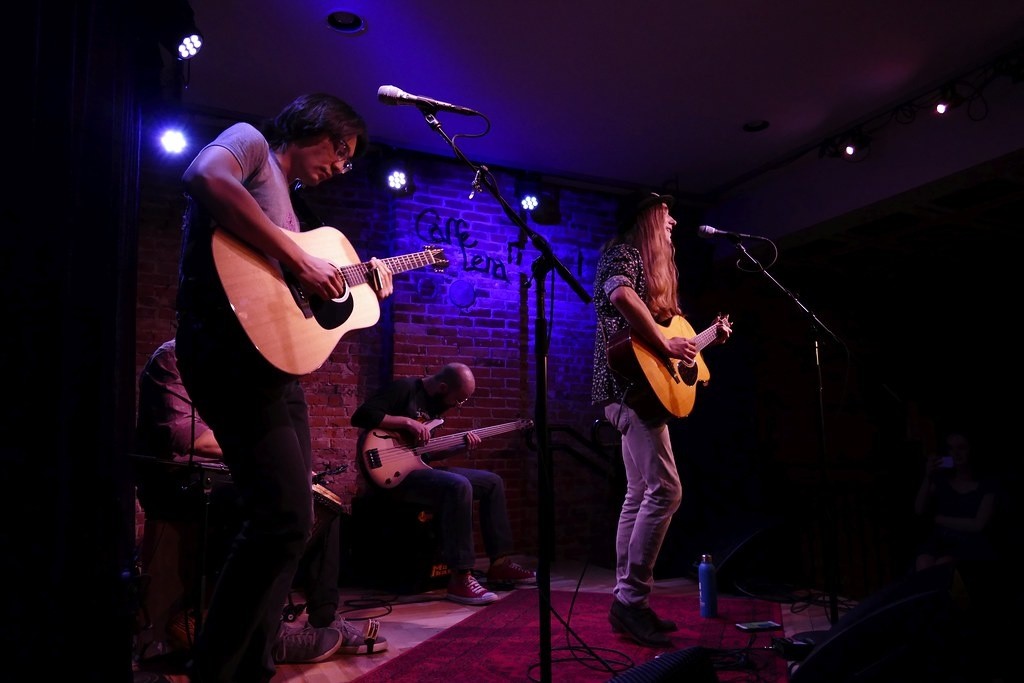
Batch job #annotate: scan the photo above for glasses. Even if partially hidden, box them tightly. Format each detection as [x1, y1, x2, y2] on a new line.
[328, 131, 352, 175]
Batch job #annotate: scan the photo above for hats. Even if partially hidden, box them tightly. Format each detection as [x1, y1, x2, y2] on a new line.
[616, 191, 674, 242]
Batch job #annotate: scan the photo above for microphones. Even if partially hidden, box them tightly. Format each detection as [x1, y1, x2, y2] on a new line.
[698, 226, 765, 241]
[377, 85, 480, 117]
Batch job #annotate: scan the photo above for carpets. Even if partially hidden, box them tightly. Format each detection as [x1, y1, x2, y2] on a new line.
[349, 590, 792, 683]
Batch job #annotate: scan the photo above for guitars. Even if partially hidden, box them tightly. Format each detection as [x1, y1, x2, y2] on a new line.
[356, 412, 535, 494]
[183, 222, 453, 389]
[606, 312, 736, 428]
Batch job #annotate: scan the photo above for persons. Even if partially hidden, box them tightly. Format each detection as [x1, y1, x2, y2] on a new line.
[171, 90, 395, 683]
[914, 425, 1010, 580]
[352, 360, 538, 603]
[592, 189, 733, 647]
[137, 336, 387, 664]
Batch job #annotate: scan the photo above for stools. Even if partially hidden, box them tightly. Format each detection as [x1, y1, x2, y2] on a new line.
[136, 489, 222, 638]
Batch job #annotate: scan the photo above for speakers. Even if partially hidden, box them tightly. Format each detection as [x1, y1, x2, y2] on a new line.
[339, 500, 457, 594]
[790, 560, 992, 683]
[688, 526, 789, 596]
[605, 644, 720, 683]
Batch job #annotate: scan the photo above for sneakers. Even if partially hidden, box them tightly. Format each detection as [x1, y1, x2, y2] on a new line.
[328, 615, 388, 654]
[446, 572, 498, 606]
[271, 621, 343, 664]
[487, 557, 537, 585]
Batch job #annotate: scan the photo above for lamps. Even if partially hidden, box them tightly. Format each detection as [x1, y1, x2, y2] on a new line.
[153, 0, 204, 62]
[511, 172, 542, 212]
[383, 157, 414, 199]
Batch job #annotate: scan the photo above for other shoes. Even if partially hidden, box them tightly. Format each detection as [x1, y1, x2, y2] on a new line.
[606, 599, 676, 646]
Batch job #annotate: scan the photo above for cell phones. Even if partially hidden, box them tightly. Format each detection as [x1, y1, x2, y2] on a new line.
[735, 621, 781, 633]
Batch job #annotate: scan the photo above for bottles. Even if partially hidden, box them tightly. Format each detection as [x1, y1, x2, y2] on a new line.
[122, 569, 138, 597]
[698, 554, 717, 617]
